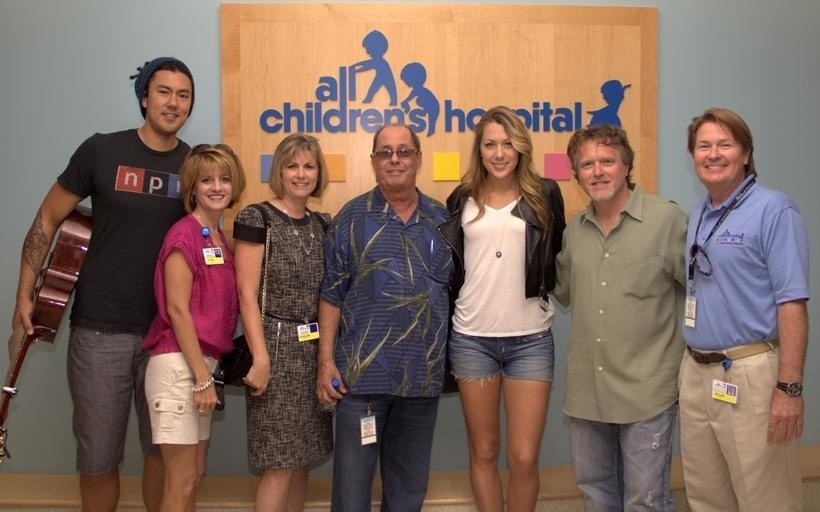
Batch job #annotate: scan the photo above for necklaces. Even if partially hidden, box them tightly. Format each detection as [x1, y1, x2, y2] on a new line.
[481, 174, 517, 257]
[278, 197, 314, 256]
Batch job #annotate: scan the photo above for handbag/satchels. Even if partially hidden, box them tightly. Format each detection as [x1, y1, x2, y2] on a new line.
[216, 333, 254, 385]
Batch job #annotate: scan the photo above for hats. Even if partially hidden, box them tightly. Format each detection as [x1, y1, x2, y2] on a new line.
[129, 57, 195, 118]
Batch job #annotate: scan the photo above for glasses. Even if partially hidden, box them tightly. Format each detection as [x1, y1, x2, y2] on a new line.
[688, 243, 713, 277]
[374, 147, 418, 159]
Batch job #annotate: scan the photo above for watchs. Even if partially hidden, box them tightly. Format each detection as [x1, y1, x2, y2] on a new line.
[776, 379, 805, 399]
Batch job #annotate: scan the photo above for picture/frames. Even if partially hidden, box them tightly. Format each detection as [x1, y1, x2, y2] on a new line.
[219, 2, 655, 244]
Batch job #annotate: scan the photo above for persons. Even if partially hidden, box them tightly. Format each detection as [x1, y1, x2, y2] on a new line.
[552, 125, 691, 511]
[445, 104, 566, 510]
[677, 109, 811, 511]
[10, 57, 196, 512]
[233, 133, 333, 512]
[142, 143, 246, 510]
[314, 124, 457, 511]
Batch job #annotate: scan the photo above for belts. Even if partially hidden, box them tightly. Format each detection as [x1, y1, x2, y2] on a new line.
[687, 341, 778, 364]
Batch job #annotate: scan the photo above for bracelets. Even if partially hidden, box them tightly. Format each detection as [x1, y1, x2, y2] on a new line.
[193, 377, 217, 394]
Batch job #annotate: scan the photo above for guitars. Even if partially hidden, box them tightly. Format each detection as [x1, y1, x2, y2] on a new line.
[1, 204, 92, 459]
